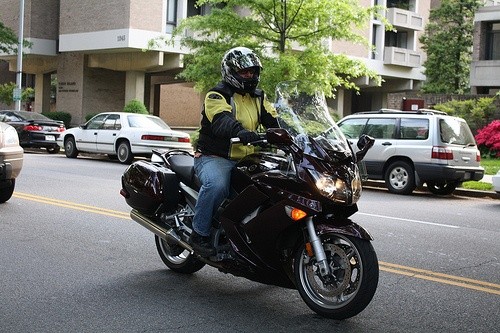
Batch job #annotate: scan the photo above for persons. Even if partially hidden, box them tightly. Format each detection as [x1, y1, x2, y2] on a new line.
[189, 47, 317, 257]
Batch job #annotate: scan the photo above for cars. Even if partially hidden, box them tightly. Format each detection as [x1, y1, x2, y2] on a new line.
[56, 112, 195, 165]
[0, 120, 24, 204]
[0, 110, 66, 154]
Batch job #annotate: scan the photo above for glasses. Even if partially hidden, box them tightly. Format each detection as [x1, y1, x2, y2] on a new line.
[238, 66, 255, 74]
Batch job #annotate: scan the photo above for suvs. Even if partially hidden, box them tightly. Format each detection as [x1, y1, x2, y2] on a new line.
[315, 108, 485, 195]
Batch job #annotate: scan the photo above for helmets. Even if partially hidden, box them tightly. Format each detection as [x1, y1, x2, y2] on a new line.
[220, 46, 262, 91]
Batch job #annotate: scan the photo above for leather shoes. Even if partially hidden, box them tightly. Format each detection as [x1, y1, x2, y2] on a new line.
[187, 229, 217, 256]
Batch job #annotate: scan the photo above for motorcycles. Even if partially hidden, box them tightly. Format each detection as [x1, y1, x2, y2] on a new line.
[120, 81, 379, 319]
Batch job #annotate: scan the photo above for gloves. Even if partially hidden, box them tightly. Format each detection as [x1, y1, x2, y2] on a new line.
[237, 129, 259, 146]
